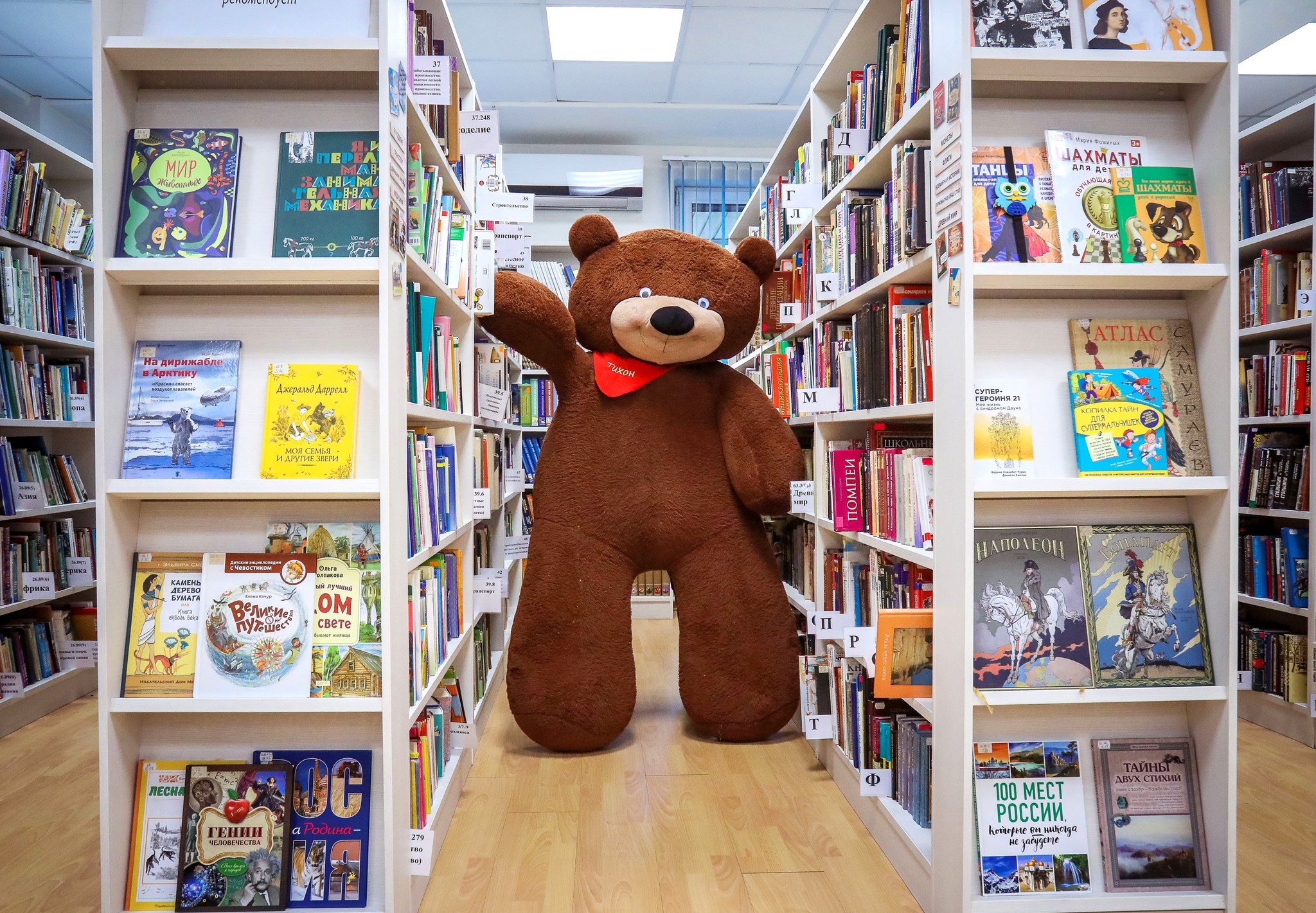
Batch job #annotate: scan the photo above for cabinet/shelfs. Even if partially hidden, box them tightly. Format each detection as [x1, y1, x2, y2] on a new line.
[523, 368, 678, 623]
[737, 0, 1241, 913]
[1239, 91, 1316, 754]
[0, 108, 98, 739]
[89, 0, 526, 913]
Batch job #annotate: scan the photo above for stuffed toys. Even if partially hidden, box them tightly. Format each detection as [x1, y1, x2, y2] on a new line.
[481, 214, 808, 754]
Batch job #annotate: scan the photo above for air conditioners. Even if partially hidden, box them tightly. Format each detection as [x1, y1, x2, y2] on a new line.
[500, 154, 644, 213]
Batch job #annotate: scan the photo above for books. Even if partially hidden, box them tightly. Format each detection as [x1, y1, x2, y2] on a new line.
[0, 341, 94, 422]
[407, 523, 493, 708]
[0, 516, 96, 606]
[0, 435, 89, 516]
[114, 126, 382, 258]
[735, 0, 934, 831]
[405, 281, 532, 427]
[409, 664, 467, 830]
[405, 423, 515, 559]
[0, 144, 94, 261]
[0, 246, 86, 340]
[473, 613, 492, 711]
[405, 0, 467, 193]
[972, 0, 1214, 896]
[118, 339, 386, 913]
[403, 124, 520, 365]
[1235, 160, 1314, 241]
[1235, 424, 1311, 702]
[497, 256, 676, 599]
[1239, 249, 1313, 329]
[0, 601, 98, 699]
[1238, 340, 1311, 418]
